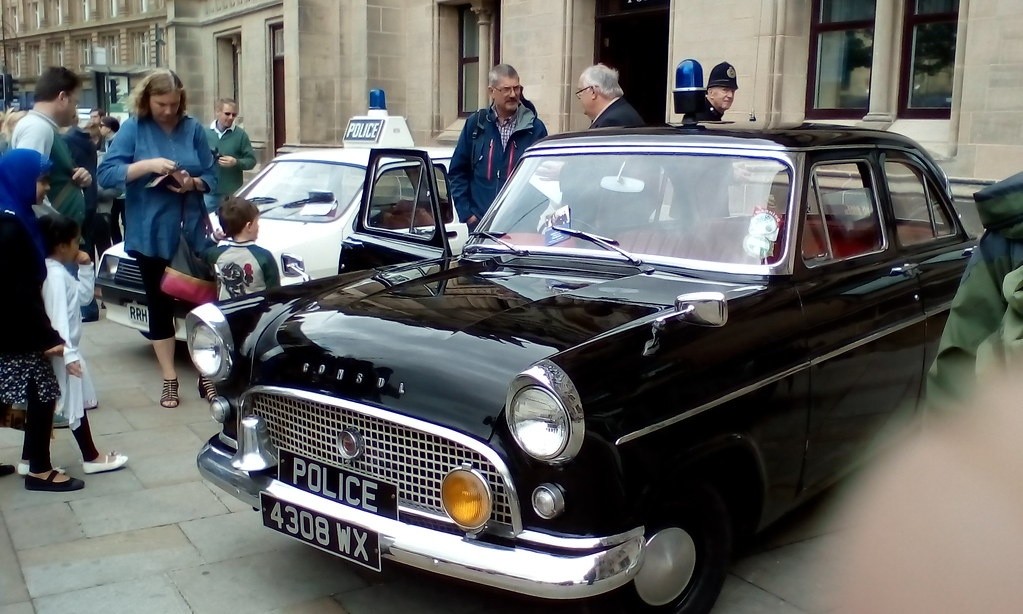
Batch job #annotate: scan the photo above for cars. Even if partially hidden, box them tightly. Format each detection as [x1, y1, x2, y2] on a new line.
[184, 127, 979, 614]
[97, 146, 681, 363]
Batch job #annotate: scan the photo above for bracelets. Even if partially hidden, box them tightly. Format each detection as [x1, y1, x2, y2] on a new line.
[191, 177, 198, 192]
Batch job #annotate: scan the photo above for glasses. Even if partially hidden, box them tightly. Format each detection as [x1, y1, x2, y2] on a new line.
[224, 112, 237, 117]
[100, 124, 106, 128]
[491, 85, 524, 96]
[576, 85, 599, 99]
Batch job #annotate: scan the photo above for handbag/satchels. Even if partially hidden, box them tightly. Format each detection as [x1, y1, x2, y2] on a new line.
[159, 231, 221, 304]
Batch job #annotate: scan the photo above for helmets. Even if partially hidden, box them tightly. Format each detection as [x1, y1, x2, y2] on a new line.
[707, 62, 738, 89]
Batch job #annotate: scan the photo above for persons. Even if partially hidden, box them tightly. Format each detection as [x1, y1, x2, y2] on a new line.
[532, 63, 658, 233]
[0, 72, 128, 491]
[449, 64, 550, 238]
[666, 61, 740, 217]
[97, 69, 220, 407]
[712, 160, 1023, 614]
[193, 198, 281, 303]
[203, 97, 257, 214]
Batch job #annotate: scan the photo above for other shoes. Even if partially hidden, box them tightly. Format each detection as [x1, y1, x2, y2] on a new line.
[54, 413, 70, 427]
[0, 465, 15, 477]
[18, 463, 66, 476]
[83, 456, 128, 474]
[25, 471, 85, 492]
[81, 315, 99, 322]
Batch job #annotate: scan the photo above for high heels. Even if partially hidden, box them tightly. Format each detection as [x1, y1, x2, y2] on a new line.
[160, 377, 180, 408]
[198, 373, 217, 404]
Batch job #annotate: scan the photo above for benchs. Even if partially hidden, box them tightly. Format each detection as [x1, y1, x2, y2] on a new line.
[604, 205, 879, 264]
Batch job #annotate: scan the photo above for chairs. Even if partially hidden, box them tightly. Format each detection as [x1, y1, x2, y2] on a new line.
[383, 199, 436, 229]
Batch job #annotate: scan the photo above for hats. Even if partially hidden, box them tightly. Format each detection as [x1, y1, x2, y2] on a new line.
[102, 117, 120, 132]
